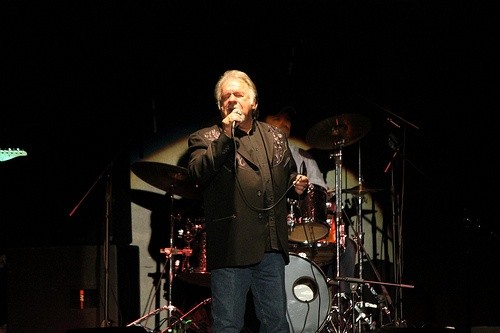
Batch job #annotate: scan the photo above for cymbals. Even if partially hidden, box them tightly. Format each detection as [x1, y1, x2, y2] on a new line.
[304, 113, 371, 149]
[130, 160, 204, 199]
[332, 185, 384, 196]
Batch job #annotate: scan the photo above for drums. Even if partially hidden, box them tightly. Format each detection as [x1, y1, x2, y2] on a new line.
[289, 184, 329, 242]
[280, 253, 333, 333]
[176, 214, 214, 288]
[299, 199, 348, 266]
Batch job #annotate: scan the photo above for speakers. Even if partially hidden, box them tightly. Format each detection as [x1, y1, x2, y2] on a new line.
[5, 244, 118, 333]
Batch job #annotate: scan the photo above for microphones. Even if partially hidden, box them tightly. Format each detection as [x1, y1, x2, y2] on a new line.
[302, 161, 307, 176]
[385, 149, 400, 172]
[231, 109, 242, 128]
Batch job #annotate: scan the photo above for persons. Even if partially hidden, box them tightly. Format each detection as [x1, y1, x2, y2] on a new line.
[259, 103, 333, 200]
[185, 70, 309, 333]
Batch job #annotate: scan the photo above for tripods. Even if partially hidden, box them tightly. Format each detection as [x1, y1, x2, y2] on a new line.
[321, 139, 394, 333]
[126, 184, 204, 333]
